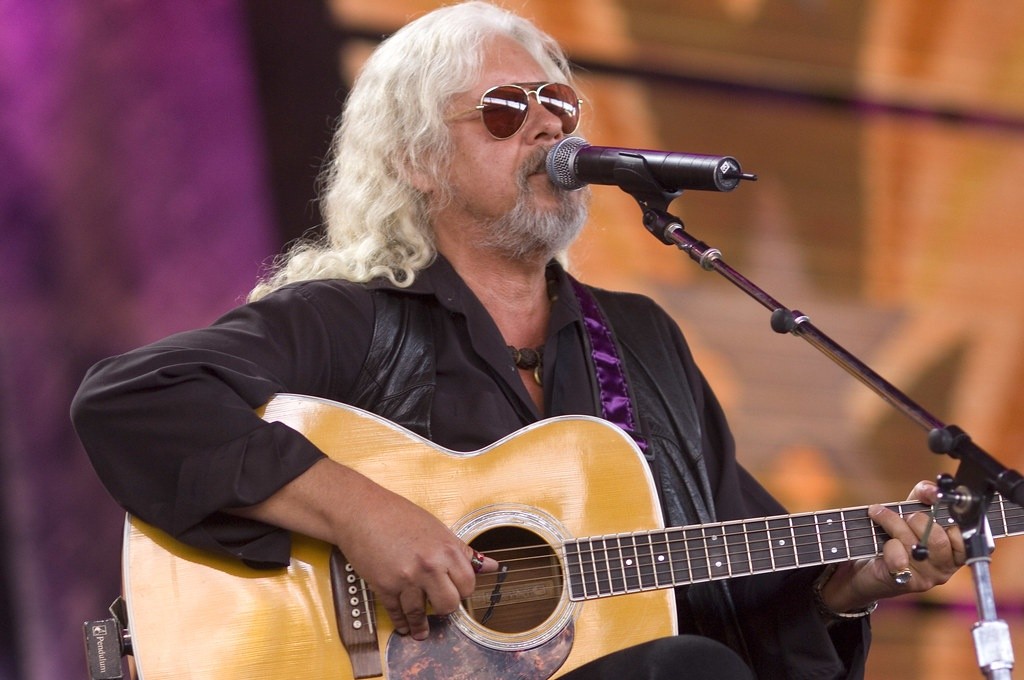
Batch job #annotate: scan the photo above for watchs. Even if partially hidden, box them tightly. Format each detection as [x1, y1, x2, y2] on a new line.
[812, 562, 878, 621]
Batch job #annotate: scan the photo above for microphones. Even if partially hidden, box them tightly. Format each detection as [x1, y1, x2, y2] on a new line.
[546, 136, 758, 193]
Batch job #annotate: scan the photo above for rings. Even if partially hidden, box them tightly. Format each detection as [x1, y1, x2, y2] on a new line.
[888, 567, 912, 584]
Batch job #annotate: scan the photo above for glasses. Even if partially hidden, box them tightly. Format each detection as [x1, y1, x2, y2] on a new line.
[443, 82, 583, 141]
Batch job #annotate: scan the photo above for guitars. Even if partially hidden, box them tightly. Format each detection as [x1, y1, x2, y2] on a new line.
[111, 389, 1024, 680]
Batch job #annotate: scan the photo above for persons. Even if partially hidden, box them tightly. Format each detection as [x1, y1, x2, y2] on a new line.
[68, 1, 968, 680]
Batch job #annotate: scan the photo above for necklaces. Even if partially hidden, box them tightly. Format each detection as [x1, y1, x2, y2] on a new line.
[507, 343, 546, 386]
